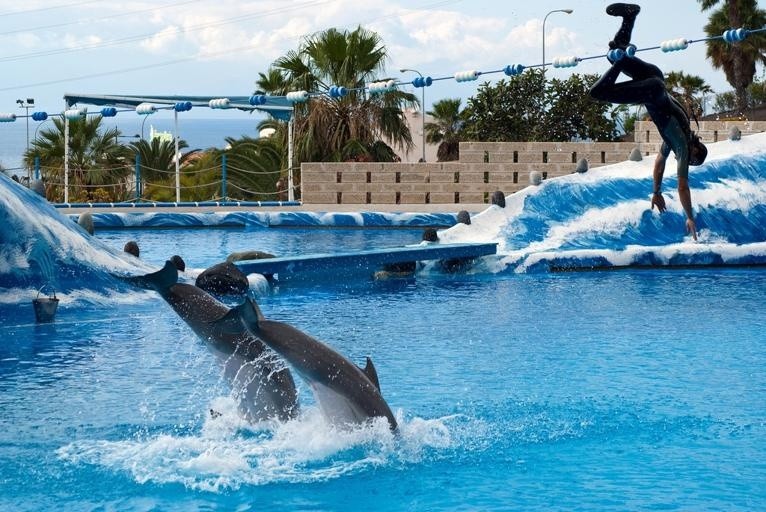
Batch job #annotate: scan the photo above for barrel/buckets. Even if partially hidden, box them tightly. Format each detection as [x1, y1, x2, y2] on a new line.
[33, 284, 61, 323]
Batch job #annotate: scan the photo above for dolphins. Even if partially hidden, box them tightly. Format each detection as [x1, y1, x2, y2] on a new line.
[218, 293, 401, 440]
[108, 259, 300, 428]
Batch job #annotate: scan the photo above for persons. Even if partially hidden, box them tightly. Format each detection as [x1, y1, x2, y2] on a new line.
[589, 3, 710, 241]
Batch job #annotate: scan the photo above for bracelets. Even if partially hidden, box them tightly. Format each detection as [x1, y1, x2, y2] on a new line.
[653, 190, 662, 196]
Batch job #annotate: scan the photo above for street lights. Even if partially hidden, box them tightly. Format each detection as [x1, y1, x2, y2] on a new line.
[399, 68, 426, 162]
[15, 97, 36, 189]
[541, 8, 574, 81]
[114, 124, 140, 144]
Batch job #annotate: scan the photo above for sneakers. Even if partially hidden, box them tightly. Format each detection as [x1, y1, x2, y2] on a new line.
[608, 41, 637, 52]
[606, 2, 640, 16]
